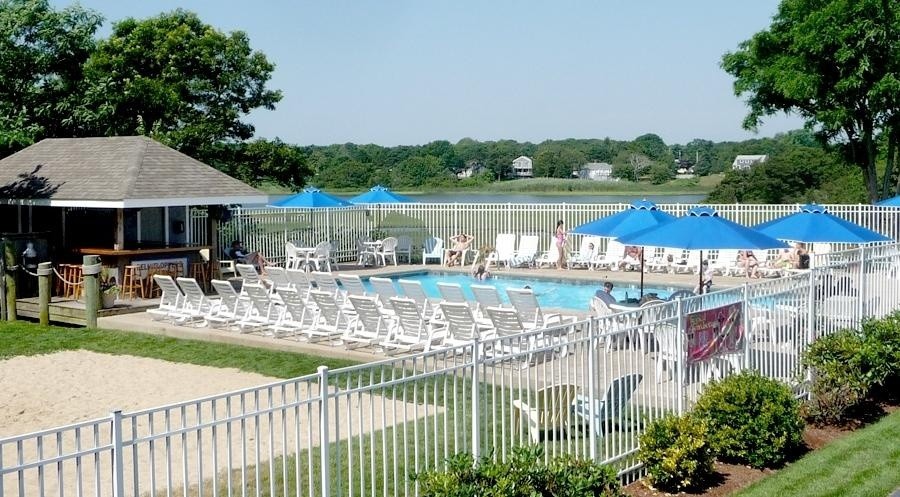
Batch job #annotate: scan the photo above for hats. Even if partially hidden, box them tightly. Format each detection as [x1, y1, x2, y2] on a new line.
[232, 240, 242, 245]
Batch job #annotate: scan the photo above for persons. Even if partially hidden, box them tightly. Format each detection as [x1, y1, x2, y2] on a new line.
[875, 195, 900, 206]
[736, 250, 758, 278]
[625, 246, 642, 261]
[475, 265, 490, 280]
[693, 259, 712, 294]
[587, 242, 594, 258]
[796, 243, 809, 269]
[230, 241, 277, 275]
[596, 282, 616, 308]
[556, 220, 569, 270]
[447, 234, 474, 267]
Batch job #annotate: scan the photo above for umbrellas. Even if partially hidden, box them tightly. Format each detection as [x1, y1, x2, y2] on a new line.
[748, 205, 895, 251]
[265, 188, 354, 235]
[348, 185, 416, 232]
[613, 208, 793, 295]
[568, 202, 679, 299]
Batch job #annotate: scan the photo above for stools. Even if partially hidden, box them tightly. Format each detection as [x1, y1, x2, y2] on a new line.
[54, 261, 207, 300]
